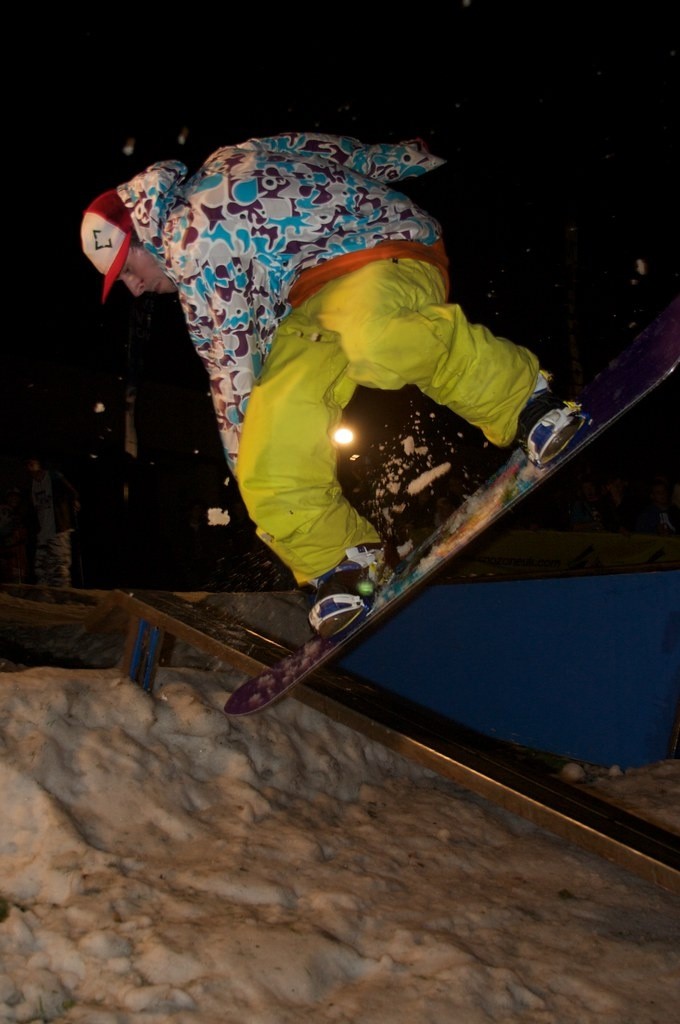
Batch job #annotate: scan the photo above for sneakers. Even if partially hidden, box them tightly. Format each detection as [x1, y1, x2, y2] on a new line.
[515, 371, 592, 469]
[309, 526, 399, 636]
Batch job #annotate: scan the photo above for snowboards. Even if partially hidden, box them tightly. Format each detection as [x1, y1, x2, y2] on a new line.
[222, 292, 677, 717]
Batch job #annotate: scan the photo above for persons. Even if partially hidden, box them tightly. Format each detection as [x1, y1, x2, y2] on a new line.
[80, 135, 591, 639]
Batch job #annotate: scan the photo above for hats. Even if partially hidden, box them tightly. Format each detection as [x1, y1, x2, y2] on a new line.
[80, 190, 133, 301]
